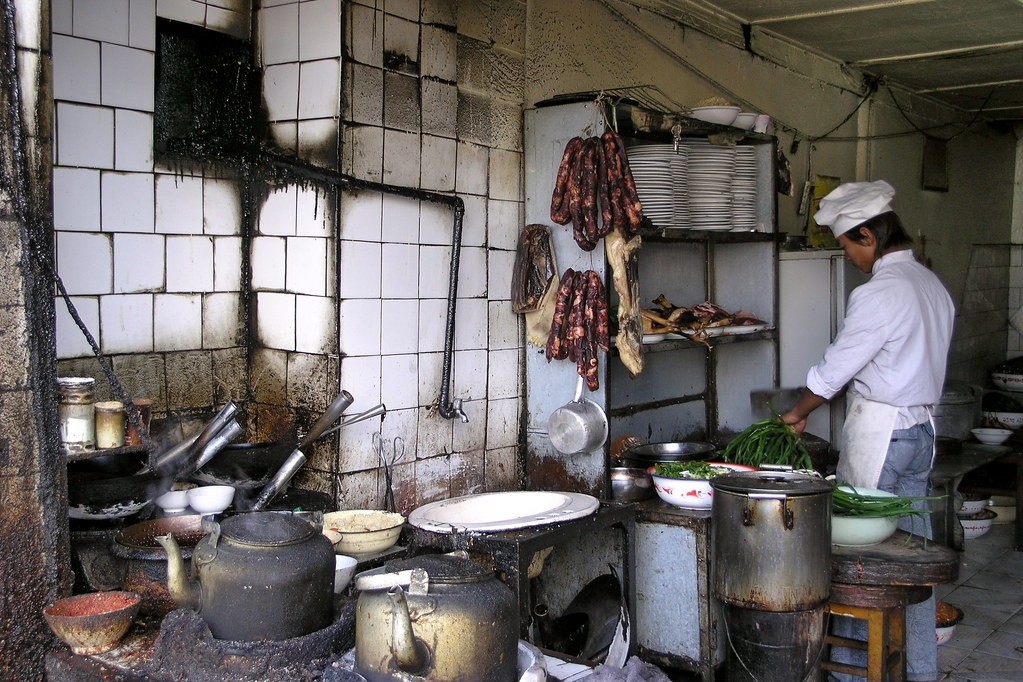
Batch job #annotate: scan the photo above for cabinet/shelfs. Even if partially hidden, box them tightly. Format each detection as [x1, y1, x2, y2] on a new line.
[523, 94, 781, 499]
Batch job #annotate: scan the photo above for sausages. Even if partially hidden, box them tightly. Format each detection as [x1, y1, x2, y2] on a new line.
[549, 131, 646, 252]
[545, 268, 610, 390]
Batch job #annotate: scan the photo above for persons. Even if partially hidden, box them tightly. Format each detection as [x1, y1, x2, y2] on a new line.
[779, 180, 955, 682]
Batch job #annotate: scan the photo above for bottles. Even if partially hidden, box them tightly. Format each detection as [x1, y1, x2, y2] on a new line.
[55, 377, 153, 450]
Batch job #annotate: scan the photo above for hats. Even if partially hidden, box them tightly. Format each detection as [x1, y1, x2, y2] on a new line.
[813, 179, 896, 238]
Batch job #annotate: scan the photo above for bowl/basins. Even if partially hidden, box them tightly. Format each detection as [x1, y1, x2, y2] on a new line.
[610, 440, 760, 509]
[831, 485, 900, 546]
[187, 484, 235, 516]
[957, 492, 1017, 539]
[333, 554, 357, 594]
[321, 510, 404, 555]
[155, 481, 197, 512]
[969, 371, 1023, 445]
[681, 105, 769, 133]
[934, 601, 964, 645]
[778, 234, 809, 251]
[43, 591, 142, 655]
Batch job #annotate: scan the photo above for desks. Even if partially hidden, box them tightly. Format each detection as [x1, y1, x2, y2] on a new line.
[396, 502, 638, 668]
[932, 441, 1023, 579]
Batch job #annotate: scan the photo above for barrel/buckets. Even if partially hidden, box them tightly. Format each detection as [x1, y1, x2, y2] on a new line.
[723, 602, 831, 682]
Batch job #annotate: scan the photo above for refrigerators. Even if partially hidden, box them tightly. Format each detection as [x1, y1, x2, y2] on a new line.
[777, 247, 873, 452]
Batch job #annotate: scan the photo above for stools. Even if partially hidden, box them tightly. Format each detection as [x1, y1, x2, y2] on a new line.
[824, 603, 907, 682]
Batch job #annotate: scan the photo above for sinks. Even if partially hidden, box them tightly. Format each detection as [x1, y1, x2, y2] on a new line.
[424, 491, 565, 528]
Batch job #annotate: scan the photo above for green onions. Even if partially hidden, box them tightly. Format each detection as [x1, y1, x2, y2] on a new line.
[654, 461, 733, 480]
[831, 481, 951, 550]
[718, 400, 813, 470]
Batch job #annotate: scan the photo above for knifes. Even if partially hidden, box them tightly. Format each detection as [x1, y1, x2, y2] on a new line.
[749, 387, 804, 425]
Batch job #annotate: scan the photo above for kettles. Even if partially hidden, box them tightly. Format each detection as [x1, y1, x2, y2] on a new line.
[353, 551, 519, 682]
[153, 506, 338, 642]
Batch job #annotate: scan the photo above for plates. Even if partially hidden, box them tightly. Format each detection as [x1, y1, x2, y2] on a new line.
[625, 137, 758, 232]
[609, 319, 769, 343]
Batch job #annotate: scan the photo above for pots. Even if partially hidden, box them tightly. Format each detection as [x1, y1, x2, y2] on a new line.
[932, 384, 975, 442]
[533, 563, 631, 669]
[187, 404, 387, 489]
[67, 400, 244, 521]
[710, 464, 836, 611]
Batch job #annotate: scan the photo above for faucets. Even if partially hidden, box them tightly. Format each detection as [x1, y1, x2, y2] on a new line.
[440, 396, 474, 423]
